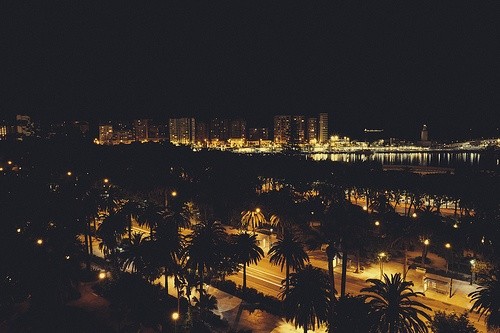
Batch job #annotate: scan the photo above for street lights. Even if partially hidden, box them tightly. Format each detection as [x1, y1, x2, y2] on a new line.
[1, 158, 264, 333]
[375, 211, 486, 299]
